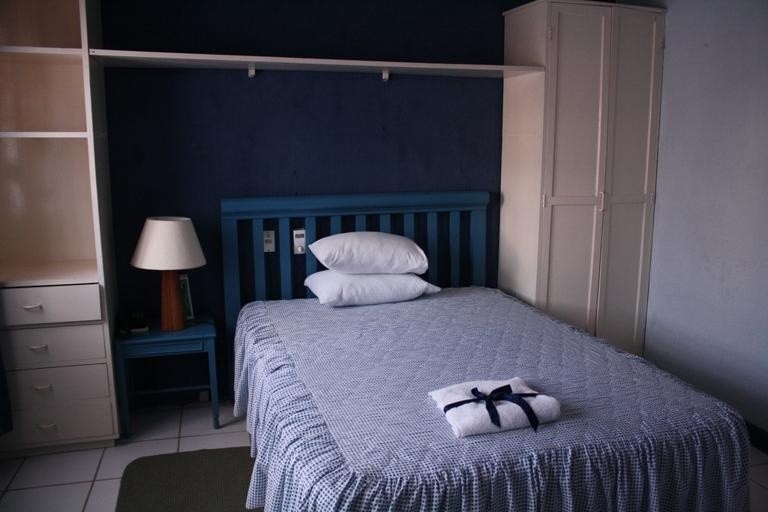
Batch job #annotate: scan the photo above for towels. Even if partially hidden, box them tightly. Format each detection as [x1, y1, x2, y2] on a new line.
[429, 375, 561, 438]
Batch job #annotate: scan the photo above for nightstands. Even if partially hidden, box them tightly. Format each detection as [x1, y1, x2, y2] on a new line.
[117, 317, 222, 438]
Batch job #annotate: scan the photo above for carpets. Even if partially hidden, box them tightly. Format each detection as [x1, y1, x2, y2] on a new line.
[118, 449, 263, 512]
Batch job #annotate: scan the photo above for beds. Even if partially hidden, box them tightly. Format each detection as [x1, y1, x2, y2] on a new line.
[223, 189, 750, 512]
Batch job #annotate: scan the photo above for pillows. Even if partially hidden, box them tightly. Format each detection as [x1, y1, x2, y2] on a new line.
[303, 231, 441, 309]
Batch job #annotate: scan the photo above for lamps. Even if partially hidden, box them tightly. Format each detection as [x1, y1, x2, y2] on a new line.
[130, 216, 207, 332]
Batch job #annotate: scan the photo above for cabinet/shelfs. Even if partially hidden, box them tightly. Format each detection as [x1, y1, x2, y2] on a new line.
[499, 2, 666, 358]
[1, 1, 122, 457]
